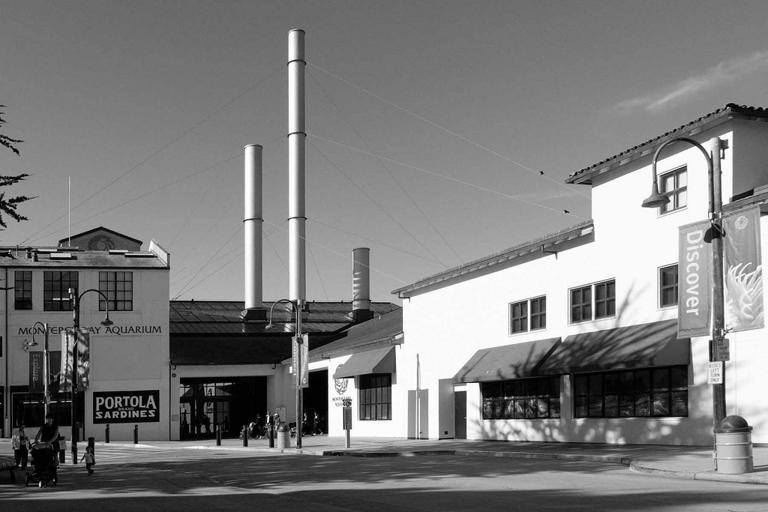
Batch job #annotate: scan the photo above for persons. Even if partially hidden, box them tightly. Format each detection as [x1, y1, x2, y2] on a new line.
[11, 412, 95, 485]
[194, 410, 323, 438]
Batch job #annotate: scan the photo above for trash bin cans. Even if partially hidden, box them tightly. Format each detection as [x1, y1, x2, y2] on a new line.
[714, 415, 753, 475]
[276, 424, 290, 448]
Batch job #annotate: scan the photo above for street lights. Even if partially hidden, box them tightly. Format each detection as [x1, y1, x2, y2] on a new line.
[69, 289, 113, 466]
[264, 298, 303, 449]
[29, 319, 50, 421]
[641, 136, 728, 432]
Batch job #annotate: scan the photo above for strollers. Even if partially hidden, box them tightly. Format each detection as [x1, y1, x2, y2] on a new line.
[23, 443, 59, 488]
